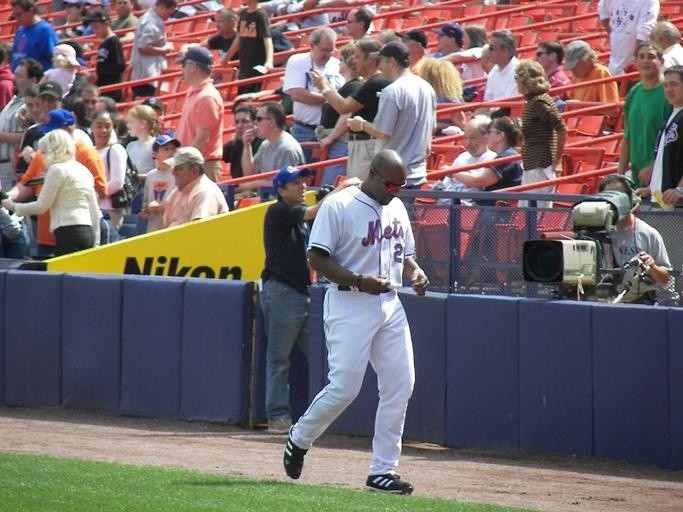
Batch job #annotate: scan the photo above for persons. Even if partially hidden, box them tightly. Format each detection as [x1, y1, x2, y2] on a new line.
[0, 1, 682, 262]
[284, 149, 430, 494]
[598, 173, 672, 306]
[259, 165, 364, 434]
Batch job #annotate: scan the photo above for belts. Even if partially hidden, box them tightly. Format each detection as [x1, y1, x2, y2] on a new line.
[336, 285, 365, 292]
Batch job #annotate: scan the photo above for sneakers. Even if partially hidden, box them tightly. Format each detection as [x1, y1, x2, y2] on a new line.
[364, 473, 414, 495]
[270, 416, 295, 436]
[283, 437, 308, 479]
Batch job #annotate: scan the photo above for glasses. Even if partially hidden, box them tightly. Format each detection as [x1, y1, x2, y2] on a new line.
[484, 127, 507, 135]
[313, 42, 334, 54]
[344, 19, 360, 23]
[375, 169, 407, 194]
[8, 7, 31, 19]
[254, 115, 274, 122]
[487, 46, 507, 50]
[534, 48, 553, 58]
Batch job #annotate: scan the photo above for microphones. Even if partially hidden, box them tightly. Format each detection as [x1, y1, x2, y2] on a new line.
[627, 199, 641, 214]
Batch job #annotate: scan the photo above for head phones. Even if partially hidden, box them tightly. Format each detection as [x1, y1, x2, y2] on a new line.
[599, 173, 642, 207]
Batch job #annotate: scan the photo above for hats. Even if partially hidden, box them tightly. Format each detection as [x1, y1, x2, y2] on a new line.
[163, 146, 204, 168]
[561, 40, 590, 71]
[396, 29, 427, 46]
[86, 1, 107, 7]
[151, 135, 179, 156]
[173, 46, 212, 66]
[144, 99, 165, 110]
[83, 9, 109, 25]
[35, 109, 75, 135]
[61, 0, 79, 5]
[272, 164, 310, 190]
[38, 81, 64, 100]
[431, 22, 463, 41]
[53, 44, 83, 71]
[370, 42, 409, 66]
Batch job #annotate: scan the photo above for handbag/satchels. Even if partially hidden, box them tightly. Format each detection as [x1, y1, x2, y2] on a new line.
[110, 160, 141, 208]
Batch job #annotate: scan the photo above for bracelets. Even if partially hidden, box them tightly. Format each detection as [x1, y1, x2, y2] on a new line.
[353, 273, 356, 288]
[412, 269, 425, 282]
[357, 275, 362, 288]
